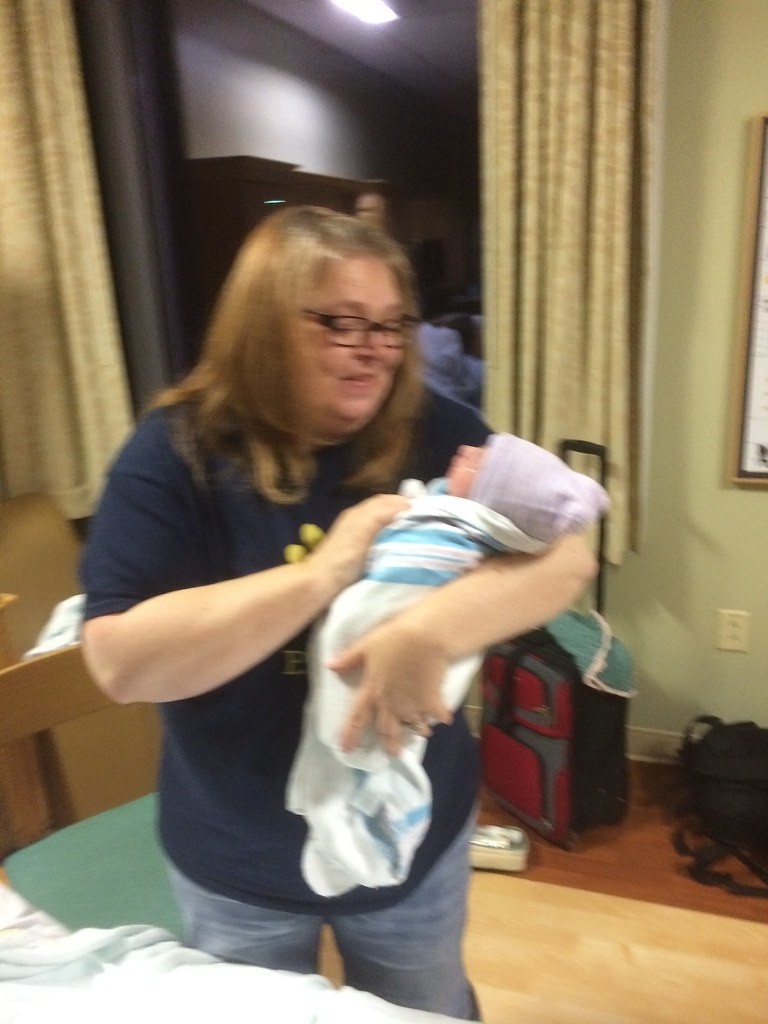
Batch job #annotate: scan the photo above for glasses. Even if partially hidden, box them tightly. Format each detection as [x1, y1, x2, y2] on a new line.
[297, 299, 421, 350]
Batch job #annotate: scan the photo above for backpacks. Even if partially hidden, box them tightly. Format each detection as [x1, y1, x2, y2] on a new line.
[668, 709, 768, 898]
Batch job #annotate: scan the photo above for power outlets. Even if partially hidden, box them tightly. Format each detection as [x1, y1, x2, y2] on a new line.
[719, 609, 751, 652]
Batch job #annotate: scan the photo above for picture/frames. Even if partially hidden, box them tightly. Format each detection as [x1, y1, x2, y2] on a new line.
[726, 110, 768, 487]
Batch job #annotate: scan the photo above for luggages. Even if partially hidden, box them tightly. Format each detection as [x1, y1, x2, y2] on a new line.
[483, 441, 635, 849]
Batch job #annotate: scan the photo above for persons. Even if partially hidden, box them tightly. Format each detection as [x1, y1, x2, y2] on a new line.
[76, 198, 601, 1024]
[289, 432, 614, 899]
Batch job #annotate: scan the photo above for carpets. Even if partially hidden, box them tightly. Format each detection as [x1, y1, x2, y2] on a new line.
[318, 868, 768, 1024]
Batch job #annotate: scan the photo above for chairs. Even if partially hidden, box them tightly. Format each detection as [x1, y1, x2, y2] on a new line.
[0, 643, 189, 948]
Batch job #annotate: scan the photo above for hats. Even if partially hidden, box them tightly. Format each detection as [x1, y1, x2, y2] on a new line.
[467, 434, 614, 537]
